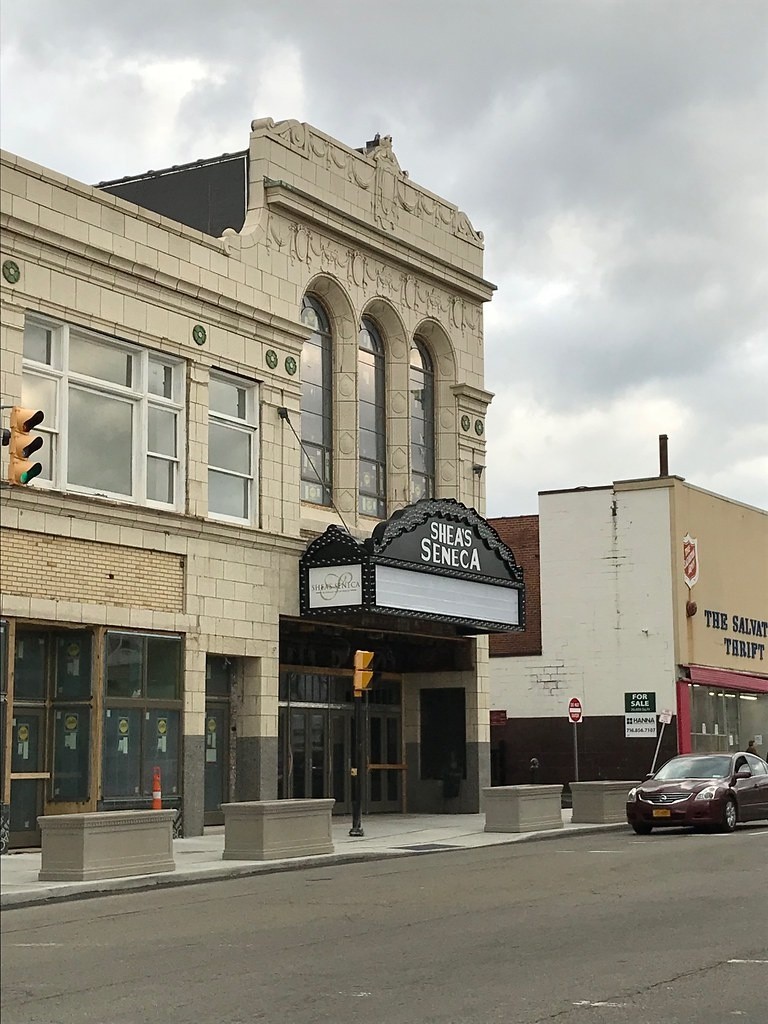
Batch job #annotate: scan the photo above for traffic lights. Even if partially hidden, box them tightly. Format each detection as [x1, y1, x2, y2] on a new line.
[353, 649, 375, 692]
[8, 405, 44, 486]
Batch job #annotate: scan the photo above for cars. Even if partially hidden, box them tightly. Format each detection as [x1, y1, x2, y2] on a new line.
[625, 751, 768, 836]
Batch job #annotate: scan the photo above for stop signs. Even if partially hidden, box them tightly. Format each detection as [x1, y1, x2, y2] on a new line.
[568, 697, 583, 721]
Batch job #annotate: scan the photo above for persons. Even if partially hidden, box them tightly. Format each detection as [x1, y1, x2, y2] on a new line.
[747, 741, 757, 754]
